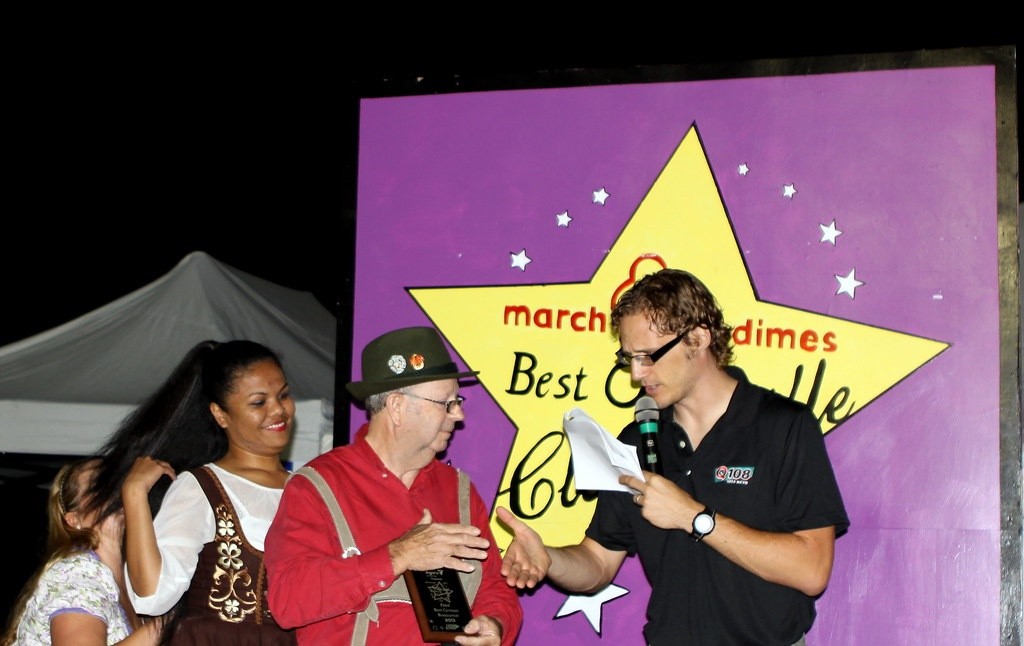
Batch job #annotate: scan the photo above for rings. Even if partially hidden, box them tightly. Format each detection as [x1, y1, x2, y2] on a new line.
[636, 494, 642, 504]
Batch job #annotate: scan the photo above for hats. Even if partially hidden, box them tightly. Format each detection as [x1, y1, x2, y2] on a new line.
[347, 327, 483, 410]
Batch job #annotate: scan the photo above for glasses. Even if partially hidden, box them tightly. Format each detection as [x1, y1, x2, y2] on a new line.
[384, 391, 466, 416]
[615, 325, 693, 366]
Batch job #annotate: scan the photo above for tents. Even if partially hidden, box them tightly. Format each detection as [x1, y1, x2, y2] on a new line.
[0, 252, 337, 470]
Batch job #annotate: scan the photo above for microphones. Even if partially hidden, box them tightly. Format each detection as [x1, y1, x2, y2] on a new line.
[629, 396, 660, 474]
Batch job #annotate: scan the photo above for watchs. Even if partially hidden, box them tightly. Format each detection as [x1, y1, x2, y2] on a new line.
[689, 503, 717, 543]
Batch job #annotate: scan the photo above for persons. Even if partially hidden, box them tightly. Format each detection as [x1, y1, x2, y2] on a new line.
[497, 269, 850, 646]
[3, 457, 174, 646]
[263, 326, 523, 646]
[78, 338, 296, 646]
